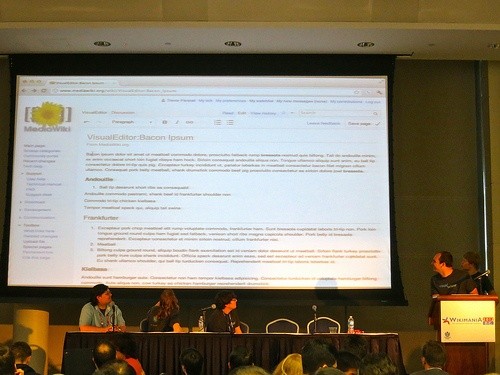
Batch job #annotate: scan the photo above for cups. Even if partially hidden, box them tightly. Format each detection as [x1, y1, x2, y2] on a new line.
[329, 327, 337, 333]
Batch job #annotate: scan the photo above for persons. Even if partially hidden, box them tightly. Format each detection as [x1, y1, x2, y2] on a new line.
[181, 349, 203, 375]
[147, 289, 180, 332]
[206, 290, 242, 334]
[408, 340, 453, 375]
[79, 283, 127, 333]
[460, 250, 499, 306]
[428, 251, 479, 323]
[0, 341, 40, 375]
[227, 345, 271, 375]
[271, 335, 398, 375]
[92, 334, 145, 375]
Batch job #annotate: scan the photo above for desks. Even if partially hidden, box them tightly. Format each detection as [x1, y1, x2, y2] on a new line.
[62, 331, 409, 375]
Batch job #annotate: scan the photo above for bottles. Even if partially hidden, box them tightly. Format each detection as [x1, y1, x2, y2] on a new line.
[199, 316, 207, 332]
[348, 316, 354, 333]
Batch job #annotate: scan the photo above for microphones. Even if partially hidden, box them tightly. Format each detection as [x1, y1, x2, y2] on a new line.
[110, 302, 116, 309]
[473, 270, 491, 281]
[201, 304, 217, 310]
[312, 305, 318, 313]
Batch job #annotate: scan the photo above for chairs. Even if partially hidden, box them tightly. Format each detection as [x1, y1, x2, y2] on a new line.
[307, 317, 341, 333]
[266, 318, 299, 334]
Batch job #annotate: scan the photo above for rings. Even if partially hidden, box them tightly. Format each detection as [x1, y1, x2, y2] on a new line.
[119, 328, 122, 331]
